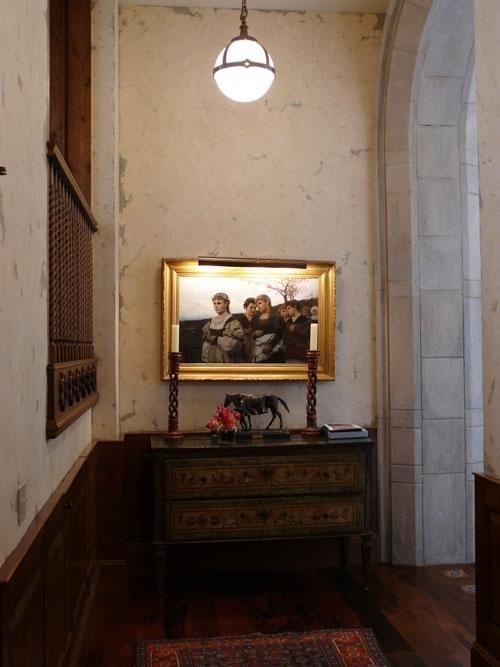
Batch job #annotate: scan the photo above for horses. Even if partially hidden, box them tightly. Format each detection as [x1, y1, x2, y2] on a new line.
[223, 391, 290, 431]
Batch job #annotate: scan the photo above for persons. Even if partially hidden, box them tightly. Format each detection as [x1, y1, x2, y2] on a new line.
[199, 292, 317, 361]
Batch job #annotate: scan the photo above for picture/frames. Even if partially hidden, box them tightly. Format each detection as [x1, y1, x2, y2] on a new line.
[160, 258, 335, 380]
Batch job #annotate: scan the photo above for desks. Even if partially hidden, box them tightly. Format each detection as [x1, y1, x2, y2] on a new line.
[150, 435, 374, 609]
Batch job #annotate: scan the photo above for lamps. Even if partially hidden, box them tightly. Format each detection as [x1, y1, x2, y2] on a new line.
[212, 1, 276, 103]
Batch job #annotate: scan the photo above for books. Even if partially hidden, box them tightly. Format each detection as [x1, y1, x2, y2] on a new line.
[320, 423, 368, 439]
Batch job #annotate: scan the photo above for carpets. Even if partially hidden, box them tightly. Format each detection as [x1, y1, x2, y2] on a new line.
[135, 628, 391, 666]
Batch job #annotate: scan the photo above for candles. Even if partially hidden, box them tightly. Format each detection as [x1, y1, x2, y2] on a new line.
[309, 324, 318, 352]
[172, 323, 180, 352]
